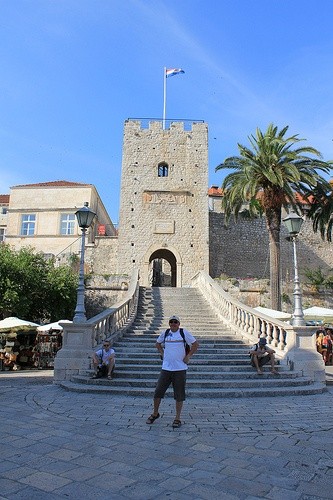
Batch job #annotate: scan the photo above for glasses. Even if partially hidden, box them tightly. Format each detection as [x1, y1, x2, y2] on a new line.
[104, 345, 109, 346]
[169, 321, 177, 323]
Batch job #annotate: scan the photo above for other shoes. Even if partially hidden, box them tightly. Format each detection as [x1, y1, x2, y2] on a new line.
[273, 371, 279, 374]
[258, 371, 264, 375]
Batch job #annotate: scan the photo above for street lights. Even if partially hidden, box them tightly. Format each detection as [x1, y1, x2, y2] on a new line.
[282, 209, 307, 327]
[72, 201, 96, 322]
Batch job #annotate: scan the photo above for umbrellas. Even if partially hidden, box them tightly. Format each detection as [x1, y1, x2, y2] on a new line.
[0, 317, 40, 332]
[37, 320, 73, 331]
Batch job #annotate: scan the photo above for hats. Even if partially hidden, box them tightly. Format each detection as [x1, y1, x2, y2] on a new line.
[169, 316, 179, 321]
[259, 338, 267, 345]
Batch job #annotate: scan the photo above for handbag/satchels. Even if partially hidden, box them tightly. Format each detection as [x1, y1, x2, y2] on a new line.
[97, 364, 108, 378]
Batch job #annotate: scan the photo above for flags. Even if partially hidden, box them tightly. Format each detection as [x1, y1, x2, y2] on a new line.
[166, 69, 185, 78]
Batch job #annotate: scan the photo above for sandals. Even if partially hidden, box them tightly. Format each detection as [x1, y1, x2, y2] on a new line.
[146, 413, 160, 424]
[107, 375, 113, 380]
[92, 374, 98, 379]
[172, 420, 181, 427]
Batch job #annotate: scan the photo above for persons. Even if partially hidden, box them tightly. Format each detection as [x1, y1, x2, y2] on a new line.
[249, 338, 278, 374]
[317, 328, 333, 365]
[146, 315, 199, 427]
[92, 341, 115, 380]
